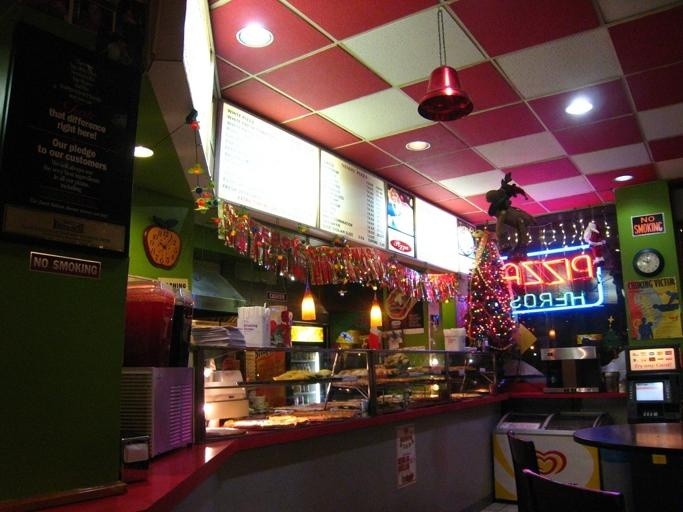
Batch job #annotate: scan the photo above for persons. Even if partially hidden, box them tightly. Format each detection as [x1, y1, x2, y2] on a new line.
[386, 186, 414, 235]
[583, 217, 609, 270]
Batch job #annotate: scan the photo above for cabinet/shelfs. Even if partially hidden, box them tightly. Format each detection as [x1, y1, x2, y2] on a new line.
[186, 344, 501, 439]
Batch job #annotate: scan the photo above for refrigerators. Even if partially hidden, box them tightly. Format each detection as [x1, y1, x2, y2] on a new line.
[287, 321, 325, 406]
[491, 411, 610, 501]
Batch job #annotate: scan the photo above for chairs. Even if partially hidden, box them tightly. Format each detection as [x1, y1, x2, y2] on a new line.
[507, 431, 624, 512]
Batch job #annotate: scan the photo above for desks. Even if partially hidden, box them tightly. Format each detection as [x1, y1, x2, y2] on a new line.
[573, 422, 683, 512]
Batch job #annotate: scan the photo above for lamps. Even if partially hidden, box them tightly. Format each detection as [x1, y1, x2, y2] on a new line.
[370, 291, 383, 326]
[419, 9, 473, 122]
[302, 265, 317, 320]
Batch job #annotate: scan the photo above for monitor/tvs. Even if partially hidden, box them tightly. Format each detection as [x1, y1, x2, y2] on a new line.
[634, 381, 665, 402]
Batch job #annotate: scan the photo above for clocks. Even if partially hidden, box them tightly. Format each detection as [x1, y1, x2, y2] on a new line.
[632, 247, 664, 278]
